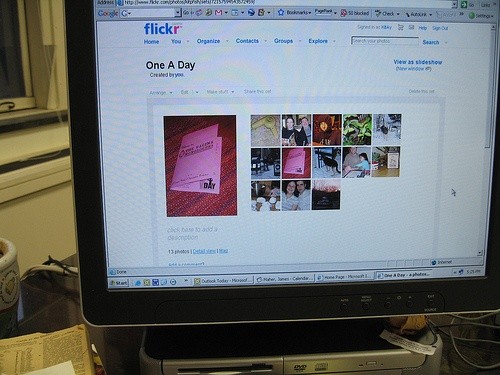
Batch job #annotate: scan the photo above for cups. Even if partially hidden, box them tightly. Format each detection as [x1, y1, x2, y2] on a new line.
[0, 238, 20, 310]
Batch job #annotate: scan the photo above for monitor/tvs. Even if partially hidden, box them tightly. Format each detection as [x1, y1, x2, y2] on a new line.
[64, 1, 500, 328]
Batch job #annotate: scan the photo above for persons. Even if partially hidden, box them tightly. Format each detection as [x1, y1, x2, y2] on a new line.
[249, 113, 409, 217]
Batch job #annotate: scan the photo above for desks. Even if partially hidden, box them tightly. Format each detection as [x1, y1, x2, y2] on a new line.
[0, 252, 143, 375]
